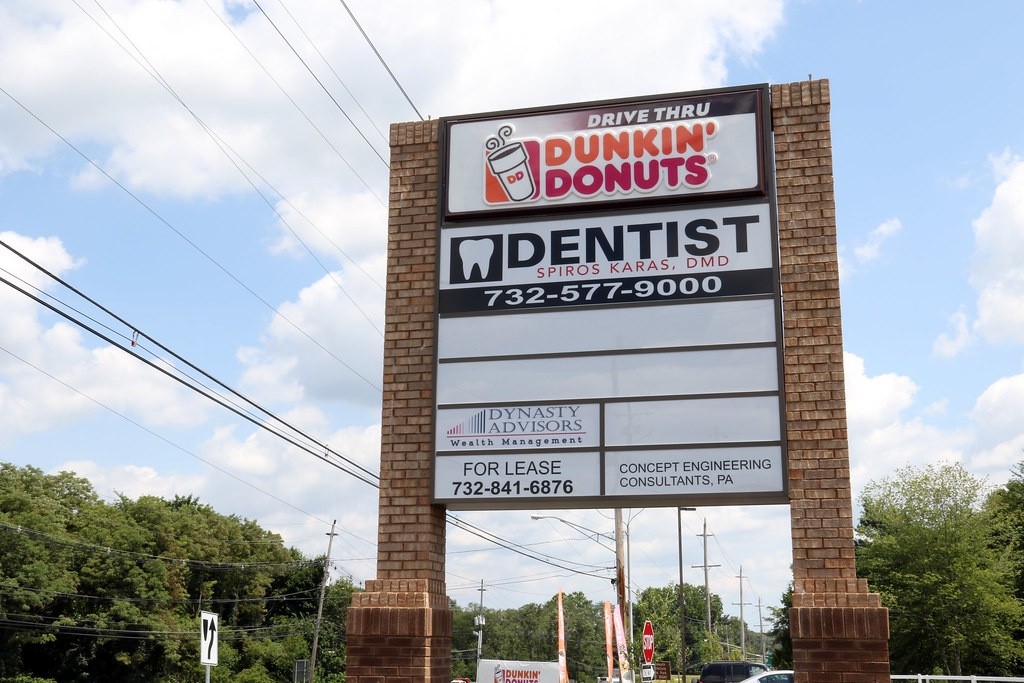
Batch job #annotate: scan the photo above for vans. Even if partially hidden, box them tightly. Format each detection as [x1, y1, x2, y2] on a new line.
[699, 662, 772, 683]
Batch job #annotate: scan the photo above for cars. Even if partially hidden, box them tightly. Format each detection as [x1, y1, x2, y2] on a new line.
[739, 670, 794, 683]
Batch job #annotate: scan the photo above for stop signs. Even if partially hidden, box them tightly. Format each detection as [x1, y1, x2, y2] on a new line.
[642, 620, 655, 664]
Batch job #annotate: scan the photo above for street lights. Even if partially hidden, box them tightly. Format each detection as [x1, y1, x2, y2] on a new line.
[529, 514, 626, 638]
